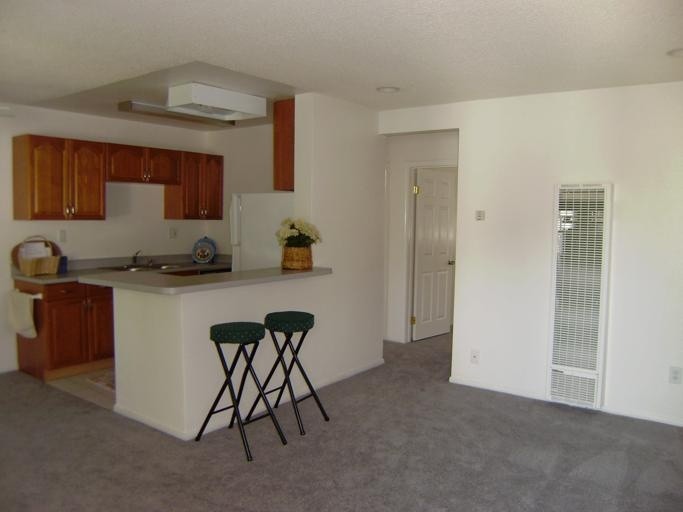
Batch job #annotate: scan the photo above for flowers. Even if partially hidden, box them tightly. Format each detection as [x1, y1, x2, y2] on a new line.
[274, 217, 322, 248]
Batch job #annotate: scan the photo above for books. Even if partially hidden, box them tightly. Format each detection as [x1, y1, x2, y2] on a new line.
[21, 240, 50, 258]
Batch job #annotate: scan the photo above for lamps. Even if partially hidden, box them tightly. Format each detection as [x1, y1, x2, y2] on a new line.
[164, 82, 266, 120]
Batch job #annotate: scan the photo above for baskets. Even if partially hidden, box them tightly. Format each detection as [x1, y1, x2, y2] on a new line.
[18, 234, 61, 275]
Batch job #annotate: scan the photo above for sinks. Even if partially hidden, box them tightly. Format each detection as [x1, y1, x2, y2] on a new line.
[106, 263, 174, 271]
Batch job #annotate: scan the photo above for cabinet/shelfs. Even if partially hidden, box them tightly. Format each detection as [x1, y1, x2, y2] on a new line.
[16, 282, 114, 383]
[108, 143, 180, 183]
[11, 134, 106, 220]
[165, 151, 223, 220]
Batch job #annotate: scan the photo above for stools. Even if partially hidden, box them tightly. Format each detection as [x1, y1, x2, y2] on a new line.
[193, 322, 287, 461]
[245, 311, 329, 436]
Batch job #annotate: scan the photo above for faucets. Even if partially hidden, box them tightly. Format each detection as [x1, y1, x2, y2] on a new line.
[132, 249, 142, 266]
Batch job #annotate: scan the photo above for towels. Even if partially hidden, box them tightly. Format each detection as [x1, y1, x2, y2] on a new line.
[7, 290, 38, 340]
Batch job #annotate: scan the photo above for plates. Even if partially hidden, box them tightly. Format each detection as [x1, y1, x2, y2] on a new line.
[192, 238, 216, 263]
